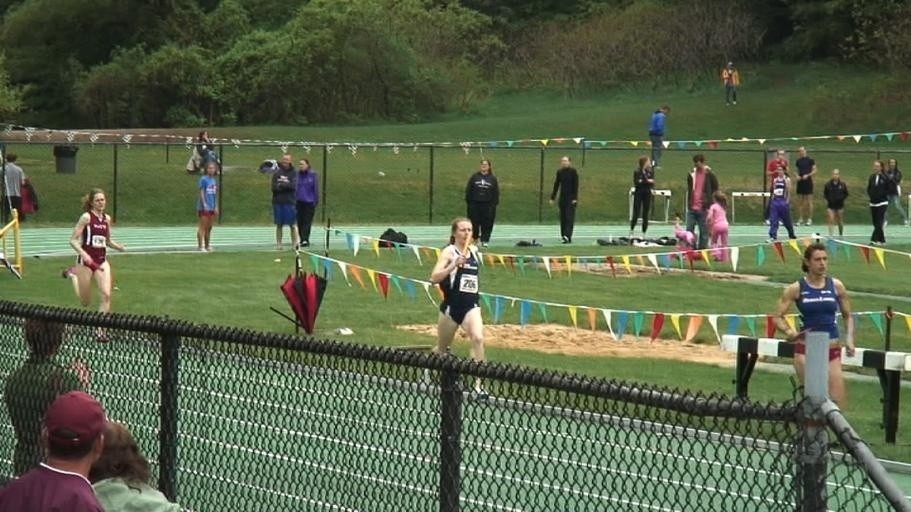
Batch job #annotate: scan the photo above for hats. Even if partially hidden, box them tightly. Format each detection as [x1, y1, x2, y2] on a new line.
[46, 390, 106, 445]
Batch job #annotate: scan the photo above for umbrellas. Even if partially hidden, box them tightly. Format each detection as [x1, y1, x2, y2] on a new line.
[278, 256, 328, 337]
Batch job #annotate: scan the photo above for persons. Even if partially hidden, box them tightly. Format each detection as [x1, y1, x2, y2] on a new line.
[767, 241, 858, 415]
[418, 214, 493, 404]
[61, 185, 129, 346]
[86, 418, 187, 512]
[1, 388, 110, 512]
[4, 317, 89, 479]
[722, 59, 742, 105]
[270, 153, 298, 253]
[196, 162, 221, 254]
[0, 150, 33, 224]
[545, 154, 581, 246]
[647, 105, 672, 172]
[463, 156, 503, 248]
[195, 131, 224, 176]
[296, 157, 320, 248]
[625, 144, 905, 264]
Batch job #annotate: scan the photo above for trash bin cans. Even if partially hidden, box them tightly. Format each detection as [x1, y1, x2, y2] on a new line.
[53, 145, 79, 174]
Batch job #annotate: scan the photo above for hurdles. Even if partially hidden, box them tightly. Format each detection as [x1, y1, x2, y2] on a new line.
[732, 192, 770, 224]
[629, 189, 673, 225]
[719, 333, 910, 446]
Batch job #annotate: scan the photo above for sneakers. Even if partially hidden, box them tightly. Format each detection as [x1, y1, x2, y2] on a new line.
[63, 267, 77, 278]
